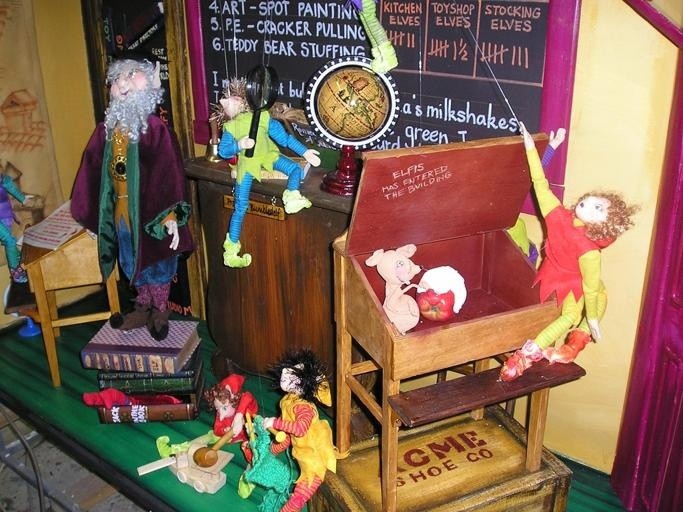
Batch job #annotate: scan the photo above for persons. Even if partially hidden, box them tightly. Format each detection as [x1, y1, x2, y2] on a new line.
[263, 347, 336, 512]
[0, 165, 34, 283]
[156, 374, 258, 498]
[70, 59, 192, 340]
[343, 0, 398, 74]
[500, 121, 640, 382]
[210, 77, 321, 268]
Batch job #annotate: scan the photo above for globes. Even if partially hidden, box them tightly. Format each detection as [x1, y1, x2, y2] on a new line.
[303, 54, 402, 199]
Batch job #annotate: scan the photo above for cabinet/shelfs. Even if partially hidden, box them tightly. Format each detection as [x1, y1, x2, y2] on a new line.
[321, 126, 587, 511]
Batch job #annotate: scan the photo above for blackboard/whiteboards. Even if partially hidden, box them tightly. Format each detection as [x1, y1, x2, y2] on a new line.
[185, 0, 582, 219]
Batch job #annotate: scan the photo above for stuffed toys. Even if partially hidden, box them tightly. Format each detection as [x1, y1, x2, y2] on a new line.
[365, 244, 427, 333]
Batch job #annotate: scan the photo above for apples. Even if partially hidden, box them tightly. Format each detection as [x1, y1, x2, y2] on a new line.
[416, 289, 455, 322]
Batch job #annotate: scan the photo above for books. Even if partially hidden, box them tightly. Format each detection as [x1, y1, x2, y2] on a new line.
[80, 314, 208, 424]
